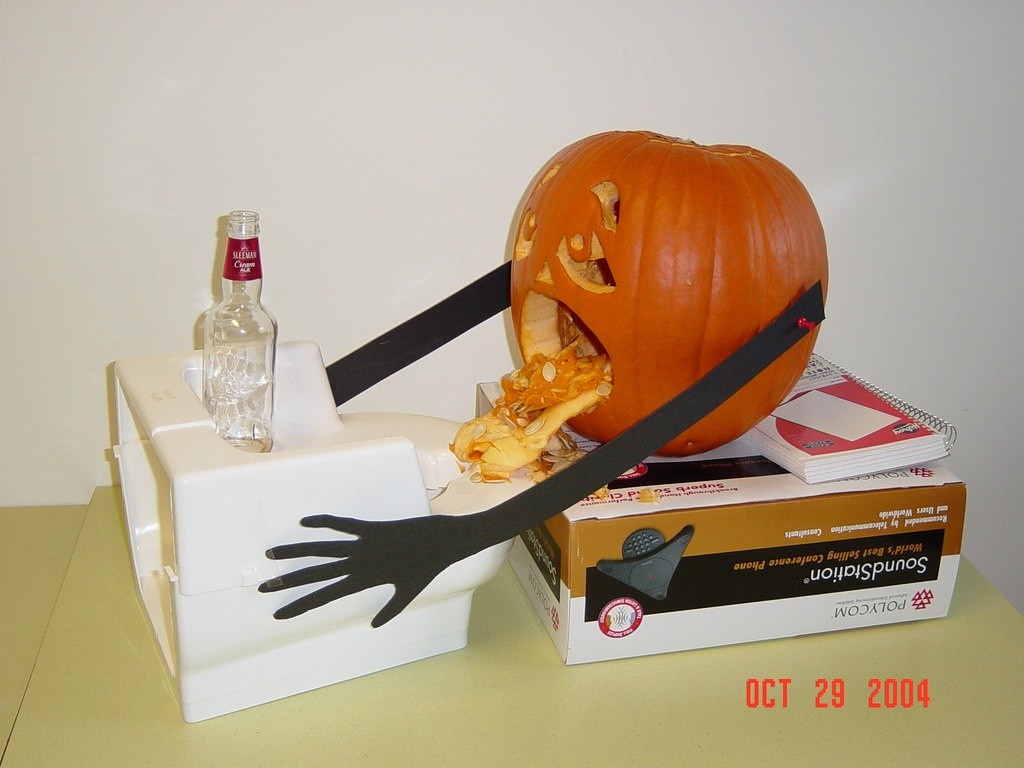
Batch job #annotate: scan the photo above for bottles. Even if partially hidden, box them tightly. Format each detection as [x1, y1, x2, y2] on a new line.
[203, 210, 278, 453]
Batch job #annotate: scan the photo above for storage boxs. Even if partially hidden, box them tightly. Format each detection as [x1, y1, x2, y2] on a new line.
[474, 382, 967, 666]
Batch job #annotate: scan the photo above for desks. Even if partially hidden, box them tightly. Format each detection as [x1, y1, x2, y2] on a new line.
[0, 484, 1023, 768]
[0, 502, 88, 763]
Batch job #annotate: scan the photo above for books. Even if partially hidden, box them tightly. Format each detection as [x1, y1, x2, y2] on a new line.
[737, 352, 957, 481]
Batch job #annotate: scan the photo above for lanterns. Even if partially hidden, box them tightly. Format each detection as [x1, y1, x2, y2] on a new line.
[509, 130, 829, 458]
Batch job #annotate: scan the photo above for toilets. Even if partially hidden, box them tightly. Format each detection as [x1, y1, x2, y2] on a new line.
[113, 341, 536, 723]
